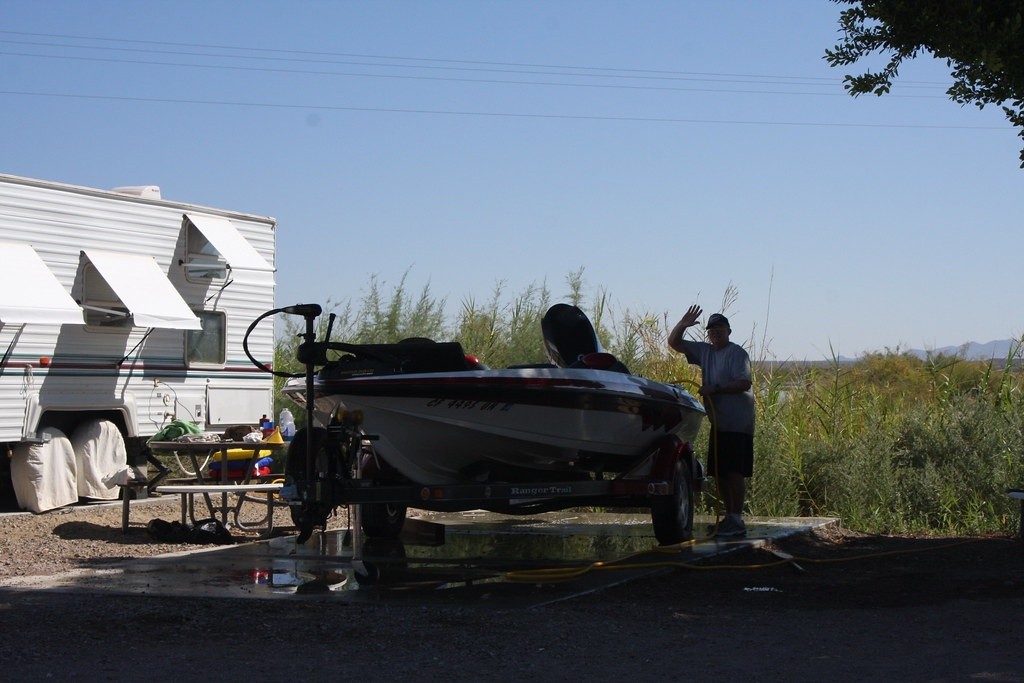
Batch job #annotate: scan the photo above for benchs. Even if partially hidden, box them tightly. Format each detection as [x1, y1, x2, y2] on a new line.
[117, 474, 327, 540]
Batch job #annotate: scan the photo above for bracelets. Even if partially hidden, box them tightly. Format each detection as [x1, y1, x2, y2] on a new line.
[715, 384, 721, 393]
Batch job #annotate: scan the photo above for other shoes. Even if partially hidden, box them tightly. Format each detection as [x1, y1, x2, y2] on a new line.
[707, 520, 747, 535]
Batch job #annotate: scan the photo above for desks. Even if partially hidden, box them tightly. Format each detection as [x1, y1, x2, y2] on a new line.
[149, 442, 292, 540]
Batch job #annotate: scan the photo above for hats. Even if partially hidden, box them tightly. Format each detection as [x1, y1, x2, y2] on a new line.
[706, 314, 728, 330]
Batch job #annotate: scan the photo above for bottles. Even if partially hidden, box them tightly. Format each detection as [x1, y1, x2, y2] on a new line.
[260, 414, 270, 431]
[279, 408, 296, 441]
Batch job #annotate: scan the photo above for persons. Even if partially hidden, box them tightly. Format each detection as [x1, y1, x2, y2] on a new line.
[668, 304, 756, 536]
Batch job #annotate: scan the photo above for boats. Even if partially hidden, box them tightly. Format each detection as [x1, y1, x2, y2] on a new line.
[278, 305, 710, 500]
[205, 422, 291, 482]
